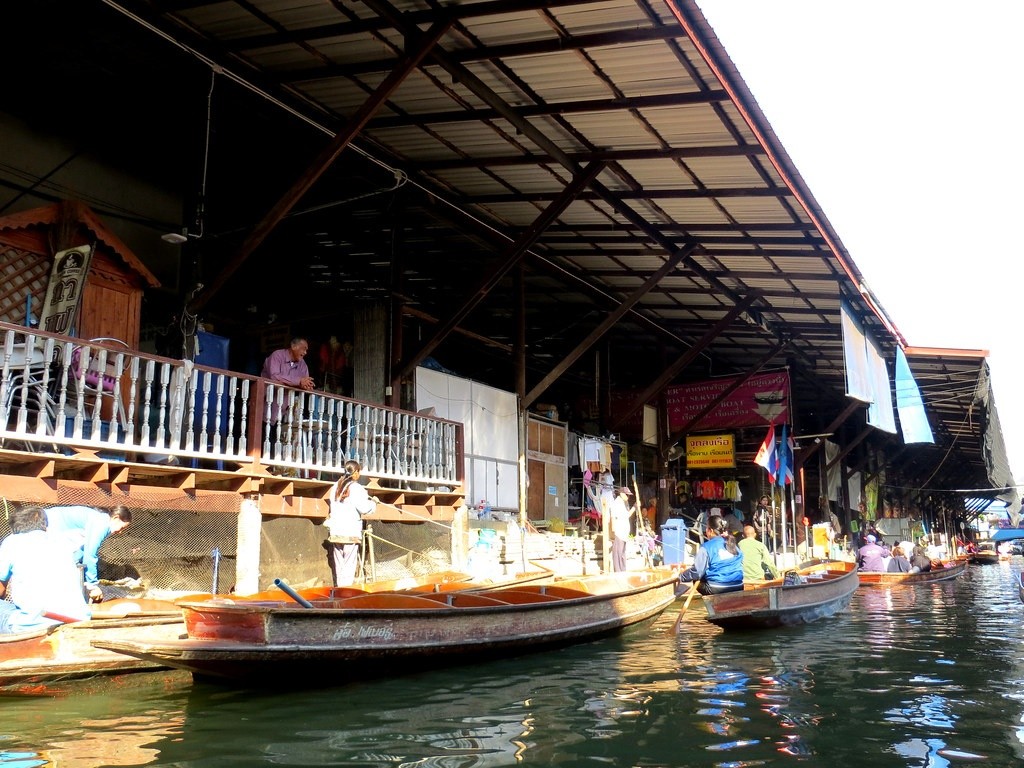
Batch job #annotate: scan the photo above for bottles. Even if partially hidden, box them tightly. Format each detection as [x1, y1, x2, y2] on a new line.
[294, 395, 299, 420]
[469, 501, 491, 521]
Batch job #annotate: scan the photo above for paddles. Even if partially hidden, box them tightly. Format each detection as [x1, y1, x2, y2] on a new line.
[40, 608, 83, 624]
[663, 580, 701, 634]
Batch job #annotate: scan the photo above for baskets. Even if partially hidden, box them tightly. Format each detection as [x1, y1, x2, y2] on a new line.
[69, 345, 118, 391]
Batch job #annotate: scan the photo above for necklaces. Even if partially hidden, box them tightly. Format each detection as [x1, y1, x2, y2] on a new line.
[754, 496, 772, 552]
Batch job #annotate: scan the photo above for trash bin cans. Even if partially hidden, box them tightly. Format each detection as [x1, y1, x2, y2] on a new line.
[660, 519, 688, 565]
[812, 524, 831, 558]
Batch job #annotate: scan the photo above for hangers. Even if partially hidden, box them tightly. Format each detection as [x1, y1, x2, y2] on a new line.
[695, 476, 738, 482]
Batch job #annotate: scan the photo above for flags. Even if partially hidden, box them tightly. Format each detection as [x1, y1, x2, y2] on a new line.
[754, 417, 779, 484]
[776, 425, 786, 486]
[784, 431, 794, 484]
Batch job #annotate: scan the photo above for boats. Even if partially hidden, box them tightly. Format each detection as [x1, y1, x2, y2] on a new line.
[700, 551, 859, 631]
[91, 555, 694, 690]
[855, 538, 1024, 606]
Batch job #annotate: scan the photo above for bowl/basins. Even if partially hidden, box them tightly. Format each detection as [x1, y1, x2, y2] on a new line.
[142, 441, 181, 465]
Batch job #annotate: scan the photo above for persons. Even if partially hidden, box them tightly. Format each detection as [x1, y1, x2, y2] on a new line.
[259, 338, 316, 460]
[633, 507, 652, 528]
[736, 525, 780, 581]
[676, 492, 693, 523]
[42, 504, 132, 600]
[679, 515, 745, 596]
[857, 535, 937, 573]
[646, 497, 657, 531]
[0, 506, 92, 635]
[721, 507, 743, 543]
[642, 480, 656, 507]
[609, 487, 641, 571]
[327, 460, 379, 587]
[693, 507, 708, 532]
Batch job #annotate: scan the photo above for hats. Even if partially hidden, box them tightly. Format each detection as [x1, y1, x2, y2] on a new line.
[863, 534, 876, 543]
